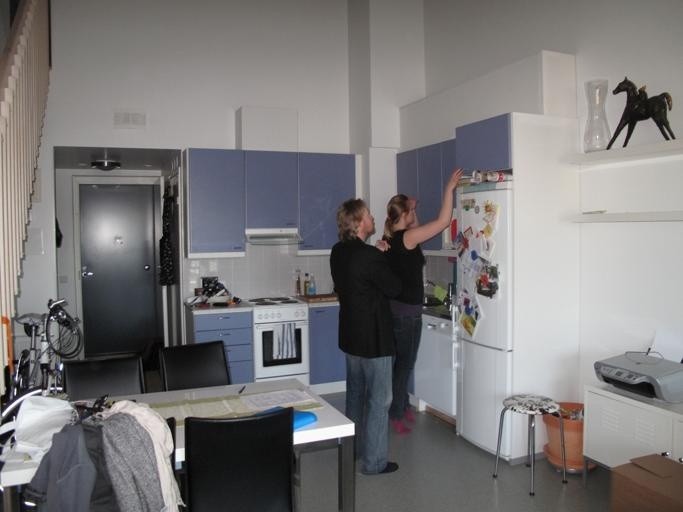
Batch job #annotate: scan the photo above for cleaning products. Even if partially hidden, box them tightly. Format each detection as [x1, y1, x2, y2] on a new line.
[306, 272, 316, 295]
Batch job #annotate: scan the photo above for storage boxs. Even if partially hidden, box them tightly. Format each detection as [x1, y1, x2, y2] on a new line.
[608, 454, 683, 512]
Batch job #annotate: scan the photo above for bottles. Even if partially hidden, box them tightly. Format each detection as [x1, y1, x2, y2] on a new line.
[294, 270, 301, 296]
[308, 273, 315, 295]
[303, 273, 308, 295]
[448, 283, 453, 305]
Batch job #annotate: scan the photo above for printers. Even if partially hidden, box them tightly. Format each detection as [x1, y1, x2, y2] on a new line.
[592, 349, 682, 406]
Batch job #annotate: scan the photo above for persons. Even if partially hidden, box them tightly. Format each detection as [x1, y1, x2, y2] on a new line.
[381, 169, 464, 434]
[328, 197, 422, 476]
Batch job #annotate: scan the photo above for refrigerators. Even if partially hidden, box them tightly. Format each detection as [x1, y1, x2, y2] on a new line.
[456, 182, 511, 467]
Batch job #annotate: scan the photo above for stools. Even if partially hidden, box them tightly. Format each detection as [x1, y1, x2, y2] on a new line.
[493, 395, 567, 495]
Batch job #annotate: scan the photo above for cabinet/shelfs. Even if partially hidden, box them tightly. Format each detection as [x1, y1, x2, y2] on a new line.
[182, 147, 246, 259]
[299, 152, 355, 256]
[183, 302, 254, 384]
[397, 142, 442, 250]
[309, 303, 347, 395]
[244, 151, 300, 228]
[456, 113, 580, 171]
[578, 136, 683, 224]
[442, 140, 456, 209]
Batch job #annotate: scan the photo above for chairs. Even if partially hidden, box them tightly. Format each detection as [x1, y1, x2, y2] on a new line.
[178, 407, 296, 512]
[159, 340, 231, 392]
[166, 416, 176, 479]
[62, 351, 147, 401]
[109, 335, 164, 371]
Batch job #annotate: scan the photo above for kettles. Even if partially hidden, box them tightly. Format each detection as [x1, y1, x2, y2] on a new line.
[440, 208, 455, 250]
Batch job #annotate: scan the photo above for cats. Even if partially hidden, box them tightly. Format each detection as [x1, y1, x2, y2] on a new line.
[199, 276, 242, 305]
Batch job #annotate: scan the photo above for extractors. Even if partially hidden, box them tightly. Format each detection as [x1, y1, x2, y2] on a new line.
[244, 228, 304, 245]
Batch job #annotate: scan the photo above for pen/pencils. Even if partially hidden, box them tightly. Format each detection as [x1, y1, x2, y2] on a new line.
[238, 385, 246, 394]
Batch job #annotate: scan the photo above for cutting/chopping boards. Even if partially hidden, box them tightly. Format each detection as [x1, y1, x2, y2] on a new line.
[298, 294, 336, 303]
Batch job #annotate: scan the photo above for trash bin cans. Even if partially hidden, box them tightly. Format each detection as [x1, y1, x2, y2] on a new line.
[543, 402, 598, 473]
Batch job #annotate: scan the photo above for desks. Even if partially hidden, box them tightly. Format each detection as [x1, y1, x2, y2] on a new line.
[0, 376, 355, 512]
[580, 382, 683, 487]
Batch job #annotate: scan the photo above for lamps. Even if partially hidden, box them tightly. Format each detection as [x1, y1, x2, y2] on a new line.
[90, 160, 120, 171]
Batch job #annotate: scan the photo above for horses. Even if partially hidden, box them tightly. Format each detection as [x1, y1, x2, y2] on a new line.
[606, 76, 676, 150]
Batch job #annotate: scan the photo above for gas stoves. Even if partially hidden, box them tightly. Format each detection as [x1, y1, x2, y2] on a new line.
[248, 297, 306, 319]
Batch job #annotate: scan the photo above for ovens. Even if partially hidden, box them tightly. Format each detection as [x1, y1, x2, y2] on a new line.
[252, 321, 310, 387]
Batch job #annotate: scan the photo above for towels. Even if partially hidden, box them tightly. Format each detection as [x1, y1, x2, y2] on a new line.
[273, 322, 297, 360]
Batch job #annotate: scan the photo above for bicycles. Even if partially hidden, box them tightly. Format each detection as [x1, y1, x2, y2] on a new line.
[10, 297, 86, 417]
